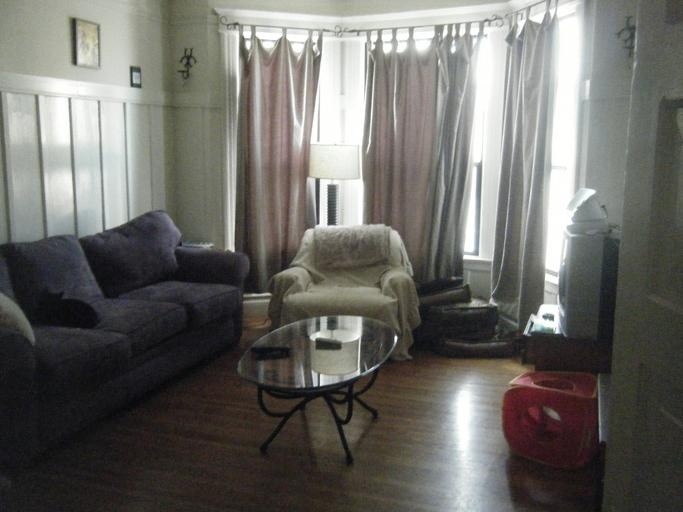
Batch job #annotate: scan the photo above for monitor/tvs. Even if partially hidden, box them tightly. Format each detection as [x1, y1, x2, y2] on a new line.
[557, 225, 620, 340]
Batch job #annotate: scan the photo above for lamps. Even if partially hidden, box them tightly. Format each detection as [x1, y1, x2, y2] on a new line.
[309, 143, 360, 226]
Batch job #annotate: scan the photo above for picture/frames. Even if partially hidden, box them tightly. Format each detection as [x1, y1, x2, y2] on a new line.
[72, 18, 100, 67]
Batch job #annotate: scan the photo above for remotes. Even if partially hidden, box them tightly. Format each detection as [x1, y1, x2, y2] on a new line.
[316, 337, 341, 349]
[250, 344, 292, 354]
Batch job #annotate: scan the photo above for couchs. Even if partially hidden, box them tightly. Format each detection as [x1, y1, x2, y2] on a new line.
[268, 224, 417, 360]
[0, 209, 249, 468]
